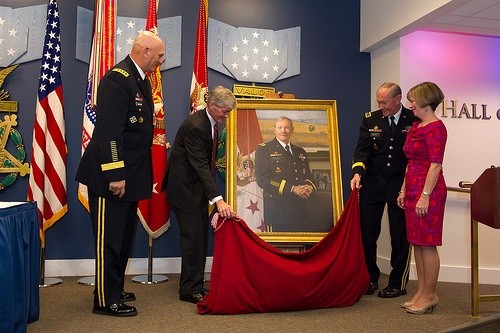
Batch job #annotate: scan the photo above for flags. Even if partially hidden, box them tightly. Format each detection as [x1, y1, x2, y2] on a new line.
[189, 0, 208, 115]
[27, 0, 68, 248]
[77, 0, 117, 212]
[137, 0, 170, 237]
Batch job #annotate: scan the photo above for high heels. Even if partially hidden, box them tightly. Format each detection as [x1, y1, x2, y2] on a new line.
[406, 295, 438, 315]
[401, 299, 414, 309]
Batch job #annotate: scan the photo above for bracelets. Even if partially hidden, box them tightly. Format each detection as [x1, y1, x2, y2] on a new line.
[400, 191, 403, 193]
[423, 192, 427, 195]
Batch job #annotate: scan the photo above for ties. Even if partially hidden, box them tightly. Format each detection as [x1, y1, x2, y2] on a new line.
[390, 116, 397, 136]
[211, 124, 217, 179]
[285, 145, 291, 155]
[144, 77, 149, 98]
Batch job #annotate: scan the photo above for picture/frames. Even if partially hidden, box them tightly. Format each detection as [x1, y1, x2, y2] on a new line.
[224, 97, 344, 244]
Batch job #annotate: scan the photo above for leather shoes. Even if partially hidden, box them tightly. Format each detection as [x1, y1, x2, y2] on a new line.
[201, 288, 209, 297]
[93, 300, 137, 316]
[180, 293, 204, 304]
[378, 287, 407, 298]
[364, 282, 379, 295]
[120, 290, 135, 301]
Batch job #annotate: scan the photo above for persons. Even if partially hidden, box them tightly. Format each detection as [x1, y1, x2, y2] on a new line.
[160, 85, 237, 305]
[74, 32, 165, 315]
[396, 81, 447, 315]
[349, 82, 417, 297]
[254, 116, 319, 232]
